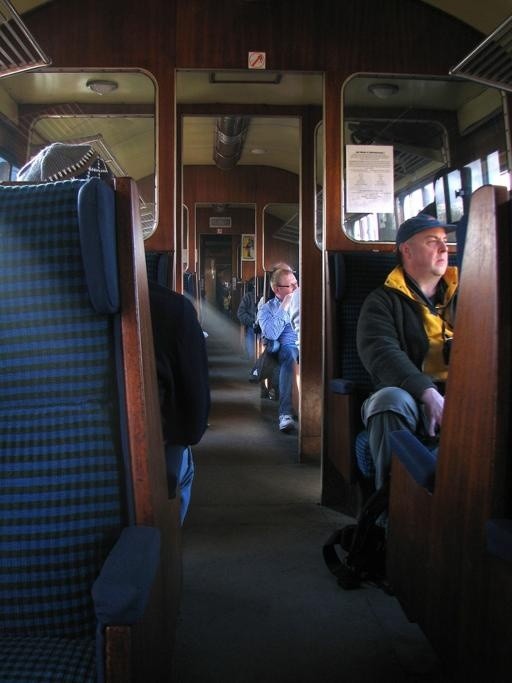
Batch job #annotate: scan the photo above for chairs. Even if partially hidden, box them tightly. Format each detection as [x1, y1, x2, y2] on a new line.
[241, 266, 301, 417]
[324, 247, 462, 523]
[377, 186, 509, 679]
[1, 175, 184, 680]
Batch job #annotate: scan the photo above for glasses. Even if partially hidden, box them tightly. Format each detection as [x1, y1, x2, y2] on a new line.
[278, 280, 297, 288]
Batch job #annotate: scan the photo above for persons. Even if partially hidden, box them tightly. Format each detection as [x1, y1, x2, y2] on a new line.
[345, 213, 462, 492]
[215, 261, 299, 432]
[138, 234, 214, 538]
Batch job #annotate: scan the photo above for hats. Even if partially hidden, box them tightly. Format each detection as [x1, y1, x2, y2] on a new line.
[396, 214, 458, 245]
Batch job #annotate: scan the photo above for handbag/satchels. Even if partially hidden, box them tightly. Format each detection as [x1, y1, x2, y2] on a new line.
[322, 483, 389, 592]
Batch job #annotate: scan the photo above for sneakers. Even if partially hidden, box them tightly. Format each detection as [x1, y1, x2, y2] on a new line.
[248, 374, 257, 382]
[279, 414, 296, 433]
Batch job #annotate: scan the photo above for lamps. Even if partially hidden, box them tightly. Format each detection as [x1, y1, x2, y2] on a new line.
[212, 116, 248, 170]
[86, 80, 119, 96]
[368, 83, 400, 102]
